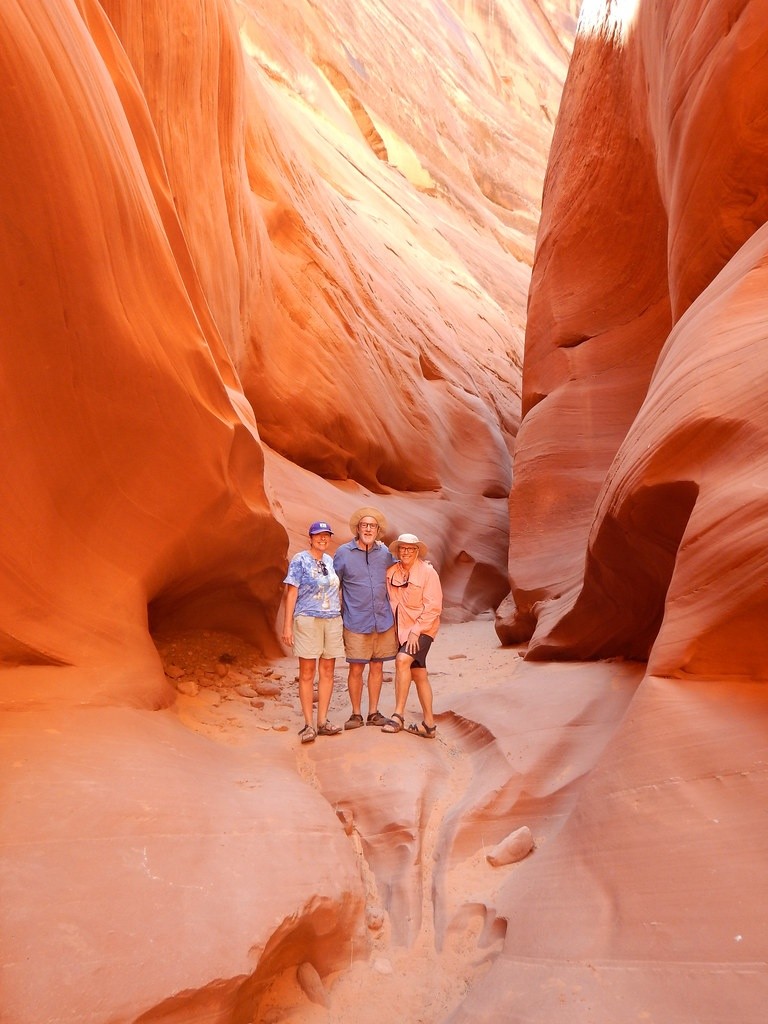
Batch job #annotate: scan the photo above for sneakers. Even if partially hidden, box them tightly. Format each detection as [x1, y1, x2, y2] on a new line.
[366, 710, 387, 726]
[345, 714, 364, 730]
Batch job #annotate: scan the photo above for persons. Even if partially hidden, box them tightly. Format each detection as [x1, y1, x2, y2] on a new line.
[381, 532, 443, 737]
[281, 521, 342, 743]
[332, 507, 433, 730]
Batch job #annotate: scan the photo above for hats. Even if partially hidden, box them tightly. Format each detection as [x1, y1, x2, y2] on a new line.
[389, 533, 428, 561]
[310, 521, 334, 536]
[349, 507, 387, 539]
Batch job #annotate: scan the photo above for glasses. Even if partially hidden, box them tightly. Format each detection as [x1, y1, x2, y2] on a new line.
[319, 559, 328, 575]
[359, 521, 378, 529]
[398, 546, 418, 552]
[390, 569, 410, 587]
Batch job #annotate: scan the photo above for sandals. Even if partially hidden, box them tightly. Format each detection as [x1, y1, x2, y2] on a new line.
[317, 719, 342, 735]
[404, 721, 436, 739]
[298, 724, 317, 744]
[381, 713, 405, 733]
